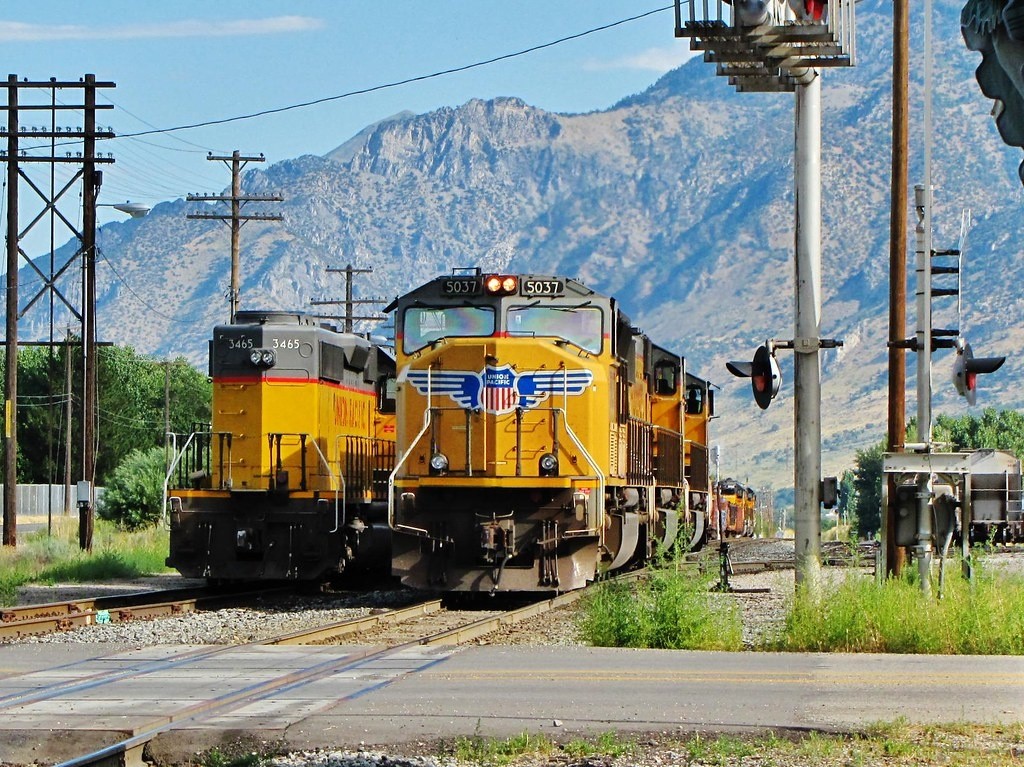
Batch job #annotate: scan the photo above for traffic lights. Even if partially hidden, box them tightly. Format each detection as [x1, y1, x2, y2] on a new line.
[726, 346, 783, 410]
[952, 343, 1006, 406]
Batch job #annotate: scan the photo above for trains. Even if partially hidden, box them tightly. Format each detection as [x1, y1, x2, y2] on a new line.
[162, 310, 397, 585]
[708, 477, 757, 539]
[376, 266, 722, 597]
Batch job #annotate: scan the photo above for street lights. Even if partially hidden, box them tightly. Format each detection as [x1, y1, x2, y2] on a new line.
[77, 202, 150, 554]
